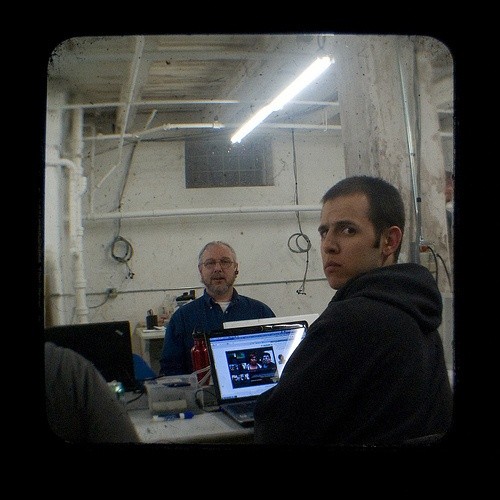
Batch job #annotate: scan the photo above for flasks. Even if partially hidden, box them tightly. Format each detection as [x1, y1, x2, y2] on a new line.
[191, 328, 210, 385]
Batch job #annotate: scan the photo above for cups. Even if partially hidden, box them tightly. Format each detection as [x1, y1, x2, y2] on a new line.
[146, 315, 158, 330]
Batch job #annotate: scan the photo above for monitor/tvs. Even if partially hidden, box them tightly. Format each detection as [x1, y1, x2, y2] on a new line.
[45, 321, 135, 392]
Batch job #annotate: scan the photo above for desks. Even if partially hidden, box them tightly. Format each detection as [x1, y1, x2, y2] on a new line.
[116, 382, 255, 444]
[135, 324, 169, 366]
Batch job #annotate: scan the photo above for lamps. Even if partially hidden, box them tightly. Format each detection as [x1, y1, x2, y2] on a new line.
[226, 35, 333, 143]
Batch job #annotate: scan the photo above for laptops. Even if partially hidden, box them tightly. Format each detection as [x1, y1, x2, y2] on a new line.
[203, 321, 309, 425]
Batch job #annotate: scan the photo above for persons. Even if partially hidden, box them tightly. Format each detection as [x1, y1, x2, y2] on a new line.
[45, 342, 139, 442]
[158, 240, 276, 386]
[261, 353, 275, 368]
[246, 353, 263, 371]
[278, 354, 286, 364]
[252, 174, 452, 445]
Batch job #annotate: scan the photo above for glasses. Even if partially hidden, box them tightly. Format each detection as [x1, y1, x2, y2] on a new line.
[201, 258, 235, 270]
